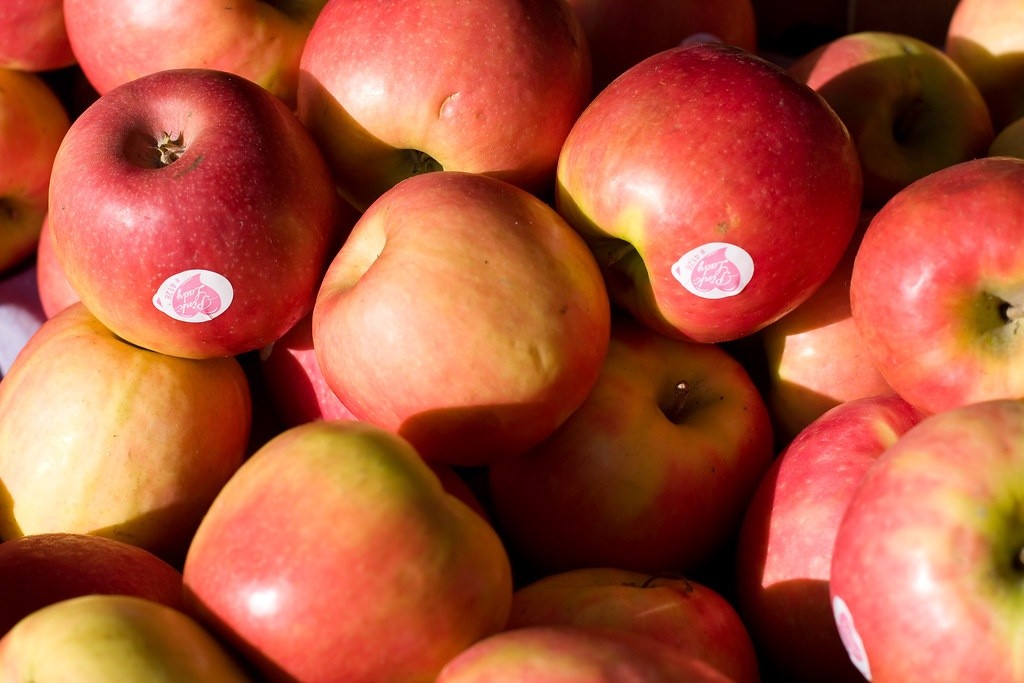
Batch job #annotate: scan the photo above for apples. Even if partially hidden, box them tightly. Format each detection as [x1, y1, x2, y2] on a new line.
[0, 0, 1024, 683]
